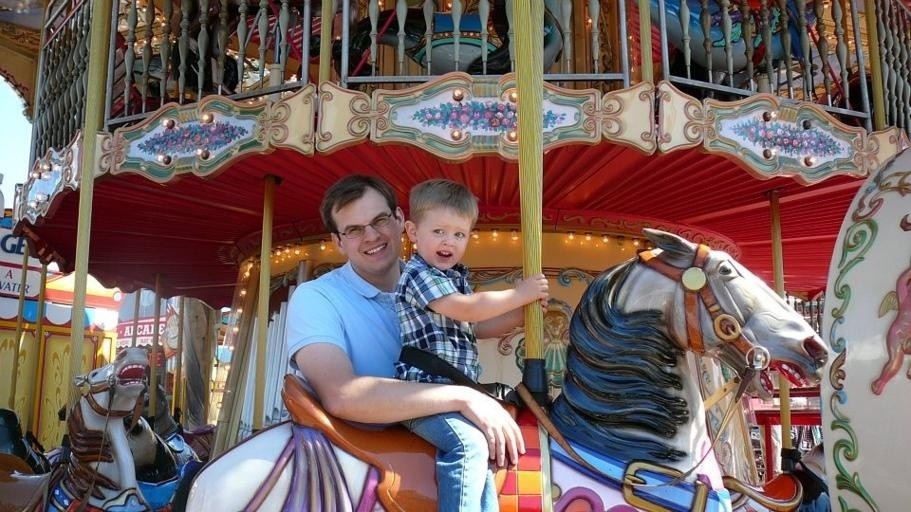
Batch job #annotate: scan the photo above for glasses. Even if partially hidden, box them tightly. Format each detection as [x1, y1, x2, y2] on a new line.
[337, 212, 392, 240]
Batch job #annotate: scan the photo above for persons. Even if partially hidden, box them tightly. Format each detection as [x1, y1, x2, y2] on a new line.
[282, 170, 526, 466]
[392, 180, 553, 512]
